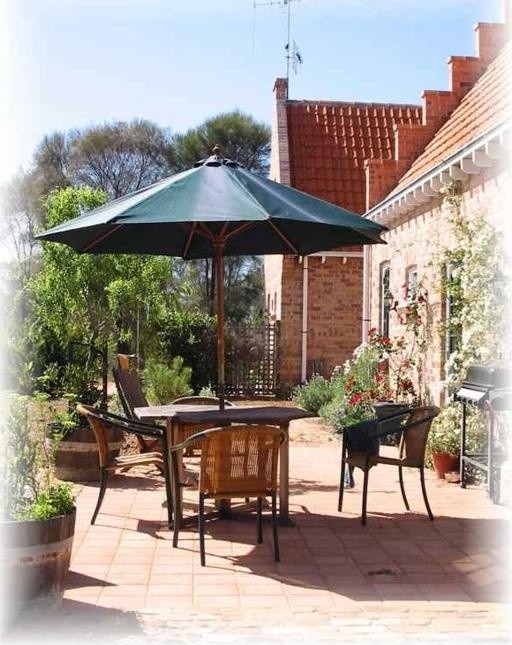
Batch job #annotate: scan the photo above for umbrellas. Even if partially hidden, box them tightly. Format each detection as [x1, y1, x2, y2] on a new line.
[33, 143, 389, 410]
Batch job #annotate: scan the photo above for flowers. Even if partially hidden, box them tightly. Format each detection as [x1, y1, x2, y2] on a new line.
[427, 401, 484, 450]
[342, 282, 429, 404]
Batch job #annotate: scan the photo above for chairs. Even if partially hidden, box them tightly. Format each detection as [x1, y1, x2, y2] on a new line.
[77, 403, 176, 532]
[112, 367, 187, 473]
[168, 396, 238, 458]
[336, 407, 441, 527]
[170, 425, 291, 567]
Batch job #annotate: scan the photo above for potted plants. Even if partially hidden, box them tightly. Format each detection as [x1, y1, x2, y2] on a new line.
[9, 185, 215, 484]
[3, 392, 79, 604]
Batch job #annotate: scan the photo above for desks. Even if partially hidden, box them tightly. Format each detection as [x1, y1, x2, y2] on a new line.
[133, 406, 319, 530]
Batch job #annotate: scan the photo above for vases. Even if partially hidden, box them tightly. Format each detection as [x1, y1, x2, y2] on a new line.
[432, 450, 460, 479]
[375, 406, 409, 447]
